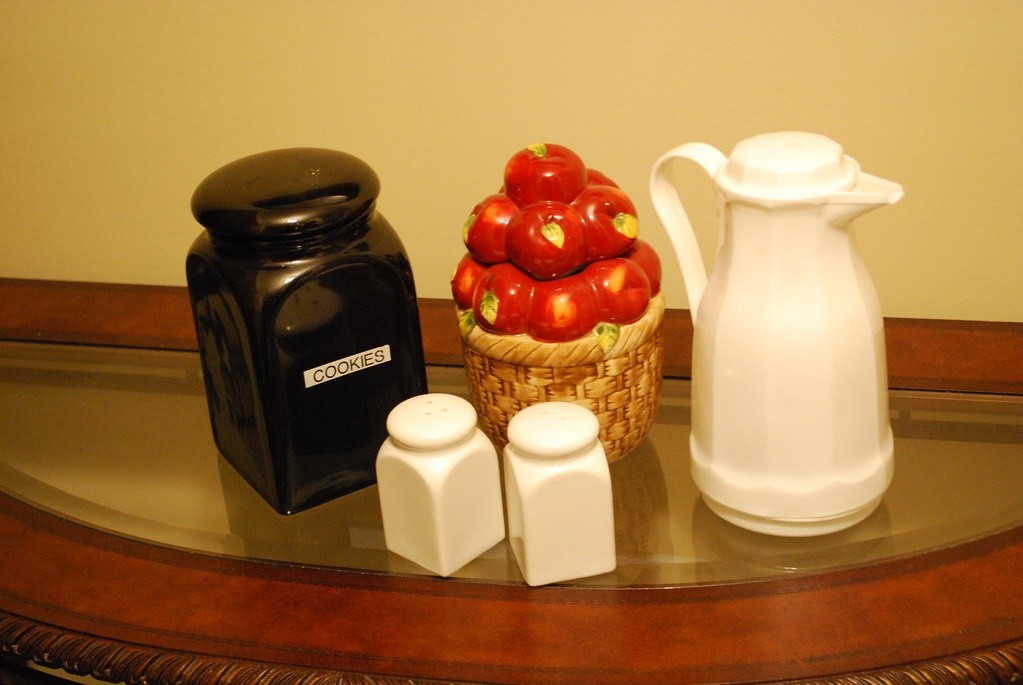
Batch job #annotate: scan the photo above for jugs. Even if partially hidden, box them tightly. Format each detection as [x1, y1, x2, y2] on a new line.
[649, 130, 906, 540]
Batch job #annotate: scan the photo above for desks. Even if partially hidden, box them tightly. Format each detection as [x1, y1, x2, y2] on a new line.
[0, 278, 1023, 685]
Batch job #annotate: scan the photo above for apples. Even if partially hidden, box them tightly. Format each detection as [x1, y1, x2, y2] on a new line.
[452, 145, 662, 343]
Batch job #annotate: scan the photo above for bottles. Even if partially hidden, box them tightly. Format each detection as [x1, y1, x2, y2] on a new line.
[376, 393, 506, 578]
[503, 401, 618, 588]
[184, 147, 430, 515]
